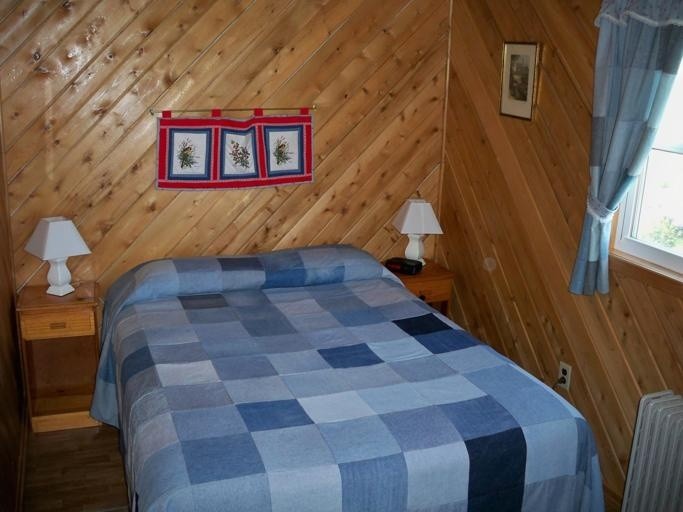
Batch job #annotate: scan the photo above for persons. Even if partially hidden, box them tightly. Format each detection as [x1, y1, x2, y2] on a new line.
[510, 53, 529, 101]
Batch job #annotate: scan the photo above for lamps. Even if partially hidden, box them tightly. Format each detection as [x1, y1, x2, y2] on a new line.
[391, 199, 443, 266]
[23, 215, 92, 297]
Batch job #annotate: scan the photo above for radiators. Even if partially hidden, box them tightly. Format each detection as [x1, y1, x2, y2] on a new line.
[619, 388, 683, 512]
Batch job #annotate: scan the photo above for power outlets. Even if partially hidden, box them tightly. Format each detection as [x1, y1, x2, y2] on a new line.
[556, 361, 572, 390]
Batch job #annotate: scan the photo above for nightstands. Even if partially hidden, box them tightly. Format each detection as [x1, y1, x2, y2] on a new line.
[382, 259, 454, 319]
[12, 281, 103, 432]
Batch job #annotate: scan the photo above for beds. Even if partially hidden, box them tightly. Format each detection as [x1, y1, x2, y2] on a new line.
[107, 277, 588, 511]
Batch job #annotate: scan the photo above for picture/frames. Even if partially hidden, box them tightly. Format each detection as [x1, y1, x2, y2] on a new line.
[499, 41, 542, 123]
